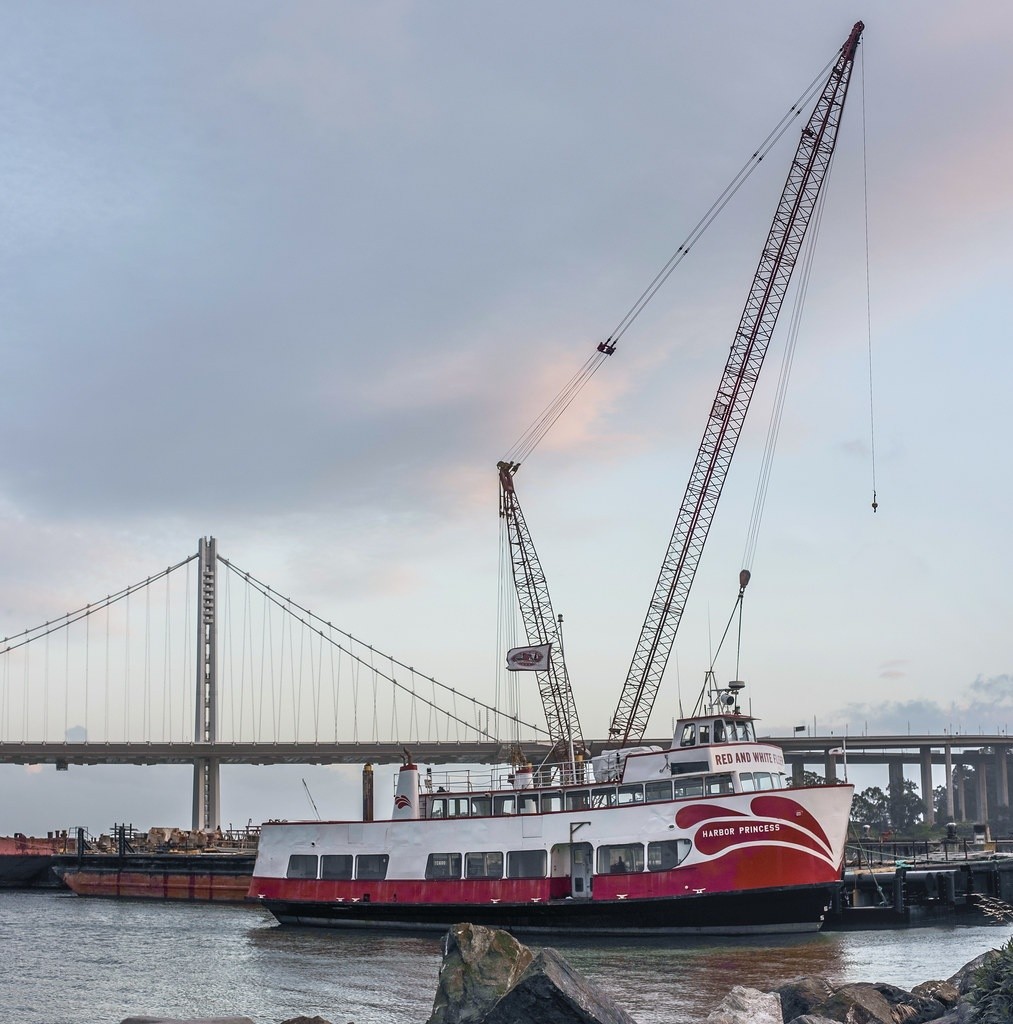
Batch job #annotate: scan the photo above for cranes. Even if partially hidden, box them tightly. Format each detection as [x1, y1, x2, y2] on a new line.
[496, 17, 870, 795]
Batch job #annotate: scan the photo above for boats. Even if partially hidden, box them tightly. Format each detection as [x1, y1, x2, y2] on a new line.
[51, 864, 252, 904]
[243, 670, 857, 938]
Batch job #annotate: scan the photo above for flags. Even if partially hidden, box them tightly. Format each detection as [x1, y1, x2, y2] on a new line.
[793, 725, 805, 732]
[505, 643, 552, 671]
